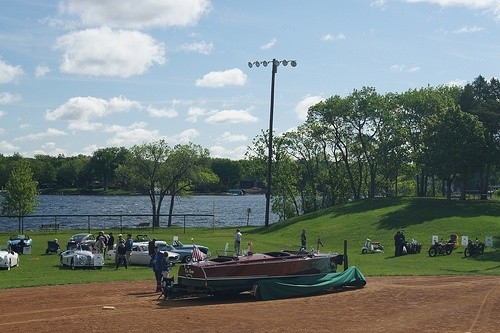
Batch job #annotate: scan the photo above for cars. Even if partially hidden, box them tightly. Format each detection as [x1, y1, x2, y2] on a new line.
[6, 234, 33, 253]
[0, 248, 20, 271]
[107, 234, 209, 266]
[66, 232, 97, 250]
[59, 249, 106, 270]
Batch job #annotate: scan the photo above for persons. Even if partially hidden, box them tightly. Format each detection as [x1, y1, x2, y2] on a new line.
[148, 238, 157, 267]
[17, 239, 27, 254]
[299, 229, 306, 251]
[234, 228, 242, 255]
[53, 239, 60, 251]
[159, 252, 173, 294]
[149, 246, 164, 292]
[394, 231, 407, 256]
[92, 231, 133, 269]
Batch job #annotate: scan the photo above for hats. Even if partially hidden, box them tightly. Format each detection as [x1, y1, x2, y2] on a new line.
[127, 234, 132, 236]
[99, 236, 105, 239]
[118, 233, 122, 237]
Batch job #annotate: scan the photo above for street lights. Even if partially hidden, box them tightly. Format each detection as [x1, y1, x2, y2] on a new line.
[247, 58, 298, 228]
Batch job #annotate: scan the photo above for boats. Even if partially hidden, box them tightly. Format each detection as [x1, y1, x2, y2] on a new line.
[177, 248, 344, 290]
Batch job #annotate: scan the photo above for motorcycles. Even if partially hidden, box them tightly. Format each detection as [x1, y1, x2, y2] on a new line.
[464, 238, 485, 258]
[428, 238, 454, 257]
